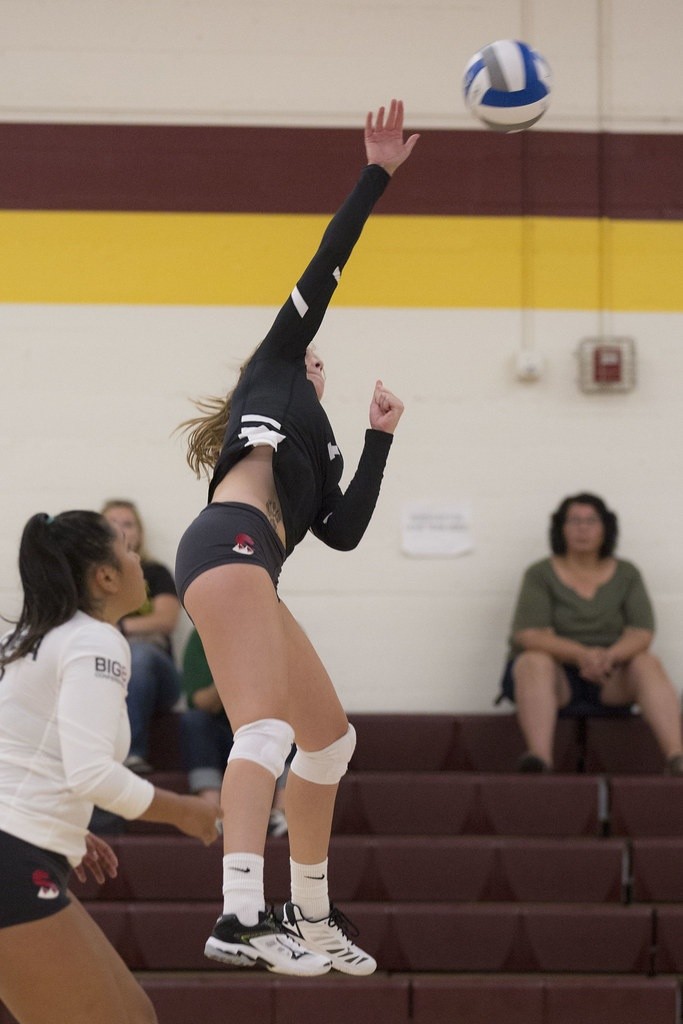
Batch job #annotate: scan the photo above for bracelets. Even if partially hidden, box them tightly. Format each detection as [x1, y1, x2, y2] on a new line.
[117, 618, 126, 636]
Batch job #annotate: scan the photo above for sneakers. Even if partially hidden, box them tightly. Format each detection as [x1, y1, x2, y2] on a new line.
[281, 901, 376, 976]
[203, 901, 333, 976]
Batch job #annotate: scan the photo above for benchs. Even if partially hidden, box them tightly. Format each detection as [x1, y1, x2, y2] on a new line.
[67, 712, 683, 1024]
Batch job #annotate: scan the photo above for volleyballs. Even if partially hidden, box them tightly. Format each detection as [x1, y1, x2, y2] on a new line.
[459, 37, 555, 135]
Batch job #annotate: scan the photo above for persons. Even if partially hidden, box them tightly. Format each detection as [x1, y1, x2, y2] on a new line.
[176, 97, 423, 979]
[0, 510, 218, 1024]
[104, 500, 181, 770]
[494, 490, 683, 776]
[179, 624, 297, 839]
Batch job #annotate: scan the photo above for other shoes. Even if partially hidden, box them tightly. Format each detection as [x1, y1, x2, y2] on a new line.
[664, 752, 683, 772]
[511, 751, 548, 774]
[266, 807, 288, 835]
